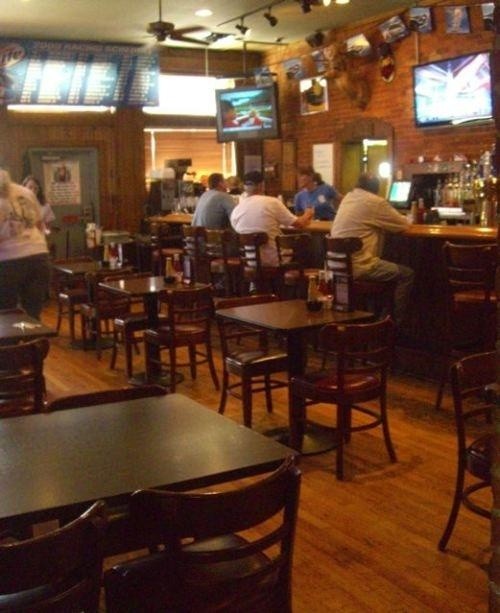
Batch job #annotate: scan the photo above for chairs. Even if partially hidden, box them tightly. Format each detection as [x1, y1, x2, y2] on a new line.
[2, 502, 107, 612]
[52, 245, 221, 393]
[2, 307, 58, 418]
[44, 385, 168, 413]
[103, 450, 303, 612]
[291, 316, 397, 478]
[437, 352, 499, 554]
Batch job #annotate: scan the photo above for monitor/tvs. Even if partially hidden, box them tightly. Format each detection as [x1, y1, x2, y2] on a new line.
[387, 179, 416, 208]
[412, 49, 495, 128]
[215, 82, 281, 142]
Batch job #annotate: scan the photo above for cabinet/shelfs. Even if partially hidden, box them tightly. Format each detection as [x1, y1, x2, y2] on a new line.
[262, 137, 298, 194]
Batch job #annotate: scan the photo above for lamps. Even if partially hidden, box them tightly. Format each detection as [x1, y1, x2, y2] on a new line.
[235, 0, 333, 37]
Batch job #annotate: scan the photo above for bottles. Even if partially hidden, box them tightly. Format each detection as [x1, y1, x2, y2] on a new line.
[308, 270, 332, 311]
[434, 144, 500, 229]
[102, 242, 118, 268]
[409, 198, 427, 225]
[165, 253, 181, 282]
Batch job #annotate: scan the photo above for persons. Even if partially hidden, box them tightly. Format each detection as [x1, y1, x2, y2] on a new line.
[1, 170, 51, 320]
[325, 171, 417, 372]
[220, 100, 265, 128]
[185, 175, 245, 292]
[292, 164, 345, 269]
[229, 170, 314, 297]
[21, 174, 57, 309]
[199, 174, 210, 193]
[225, 176, 244, 196]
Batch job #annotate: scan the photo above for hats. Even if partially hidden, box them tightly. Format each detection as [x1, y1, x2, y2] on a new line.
[243, 168, 271, 185]
[295, 165, 314, 176]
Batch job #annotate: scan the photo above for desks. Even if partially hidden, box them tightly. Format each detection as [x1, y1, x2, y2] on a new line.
[214, 299, 373, 456]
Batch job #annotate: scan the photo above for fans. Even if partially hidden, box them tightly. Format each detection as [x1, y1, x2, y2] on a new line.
[129, 0, 211, 47]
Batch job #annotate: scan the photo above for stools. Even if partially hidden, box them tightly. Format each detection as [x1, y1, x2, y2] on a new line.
[148, 222, 275, 298]
[324, 232, 397, 372]
[275, 233, 319, 299]
[435, 241, 498, 422]
